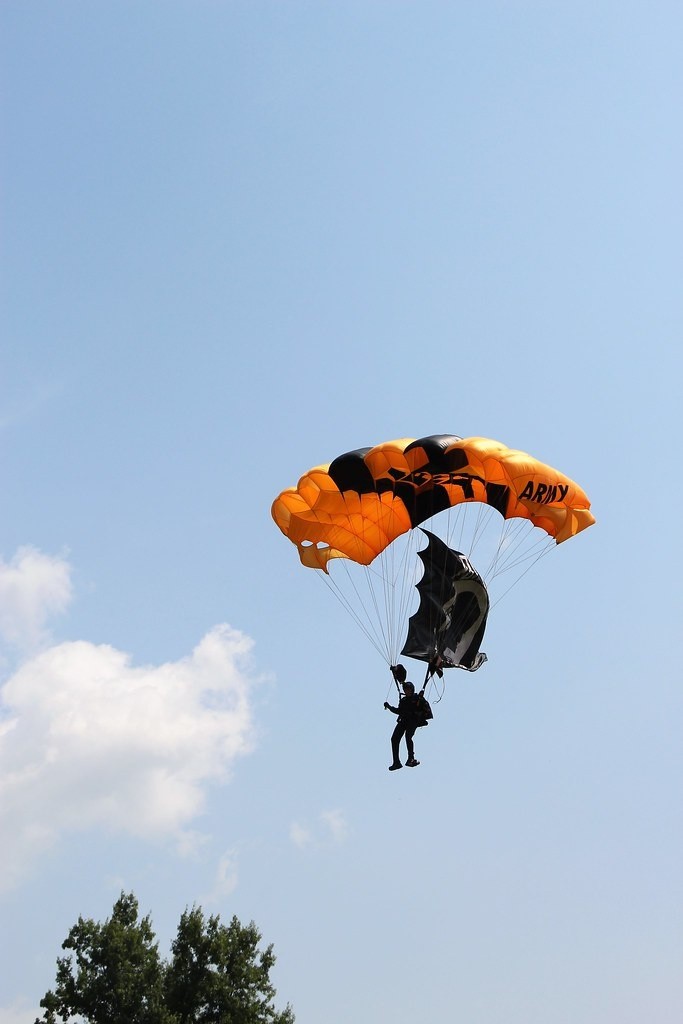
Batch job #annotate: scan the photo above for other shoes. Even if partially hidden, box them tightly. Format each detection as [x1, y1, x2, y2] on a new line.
[389, 762, 403, 770]
[405, 756, 414, 765]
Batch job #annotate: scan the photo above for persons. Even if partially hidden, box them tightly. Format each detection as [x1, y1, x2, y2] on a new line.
[384, 682, 421, 771]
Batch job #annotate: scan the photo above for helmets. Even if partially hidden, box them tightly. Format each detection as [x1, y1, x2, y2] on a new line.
[403, 682, 414, 690]
